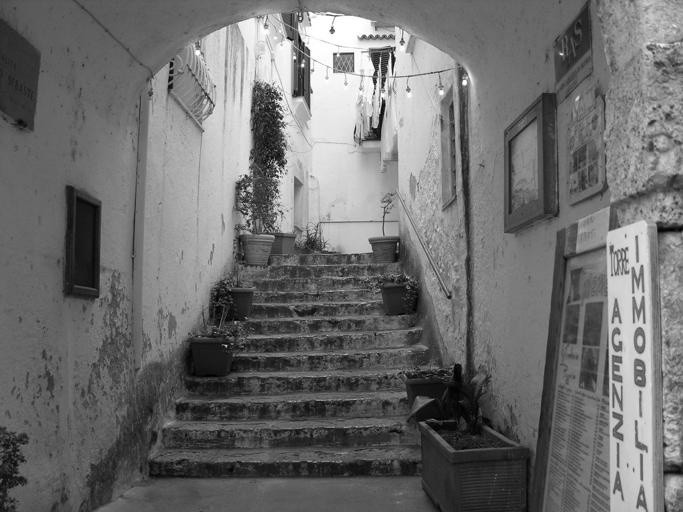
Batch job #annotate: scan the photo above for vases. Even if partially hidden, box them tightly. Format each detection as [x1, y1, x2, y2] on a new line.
[270, 234, 296, 254]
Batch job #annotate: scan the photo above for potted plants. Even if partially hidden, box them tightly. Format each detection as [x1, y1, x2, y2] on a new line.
[234, 158, 287, 265]
[368, 191, 399, 262]
[210, 275, 257, 321]
[187, 288, 241, 375]
[418, 374, 530, 512]
[377, 271, 420, 315]
[405, 369, 453, 411]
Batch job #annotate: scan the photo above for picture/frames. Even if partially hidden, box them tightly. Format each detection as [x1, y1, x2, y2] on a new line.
[504, 92, 559, 234]
[65, 185, 102, 298]
[528, 205, 618, 512]
